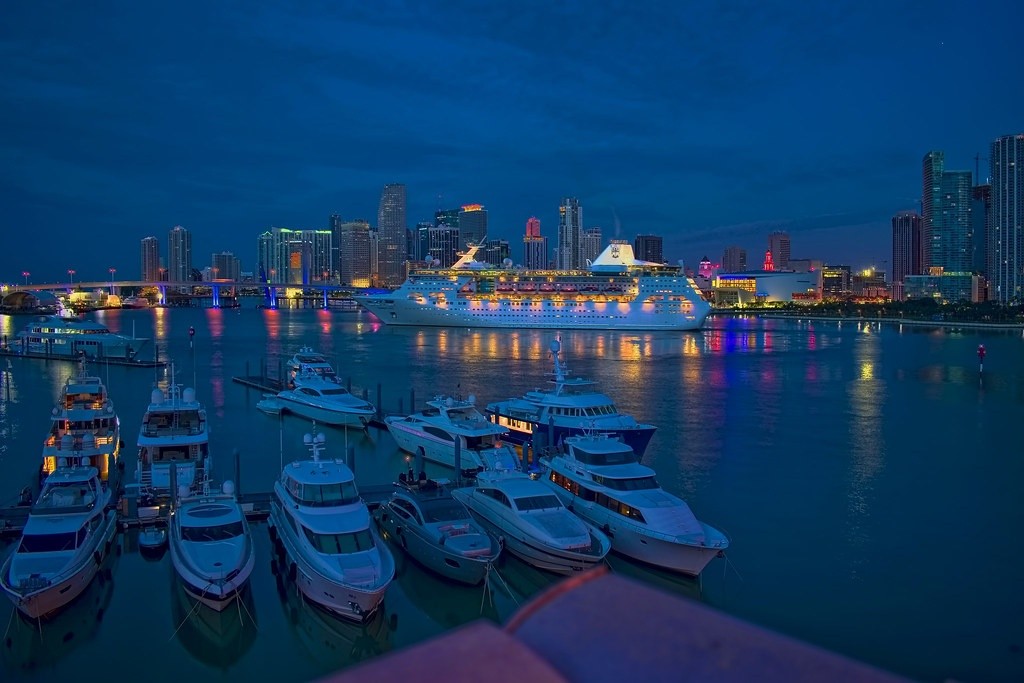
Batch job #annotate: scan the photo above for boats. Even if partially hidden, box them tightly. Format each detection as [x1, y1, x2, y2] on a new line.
[457, 288, 476, 294]
[275, 379, 378, 432]
[266, 403, 398, 625]
[603, 286, 624, 295]
[136, 360, 213, 501]
[8, 315, 151, 358]
[0, 464, 121, 617]
[556, 288, 577, 296]
[384, 383, 522, 474]
[290, 344, 344, 388]
[540, 287, 556, 295]
[485, 339, 660, 481]
[517, 287, 537, 295]
[120, 296, 149, 309]
[256, 398, 287, 417]
[39, 354, 125, 483]
[164, 473, 256, 612]
[533, 418, 732, 579]
[373, 466, 503, 585]
[580, 287, 601, 295]
[495, 287, 517, 296]
[139, 525, 167, 550]
[447, 466, 613, 576]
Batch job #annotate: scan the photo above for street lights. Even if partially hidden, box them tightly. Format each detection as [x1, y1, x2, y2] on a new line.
[66, 267, 330, 296]
[23, 270, 30, 289]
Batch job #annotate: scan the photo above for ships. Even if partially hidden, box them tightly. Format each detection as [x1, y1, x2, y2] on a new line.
[350, 260, 718, 332]
